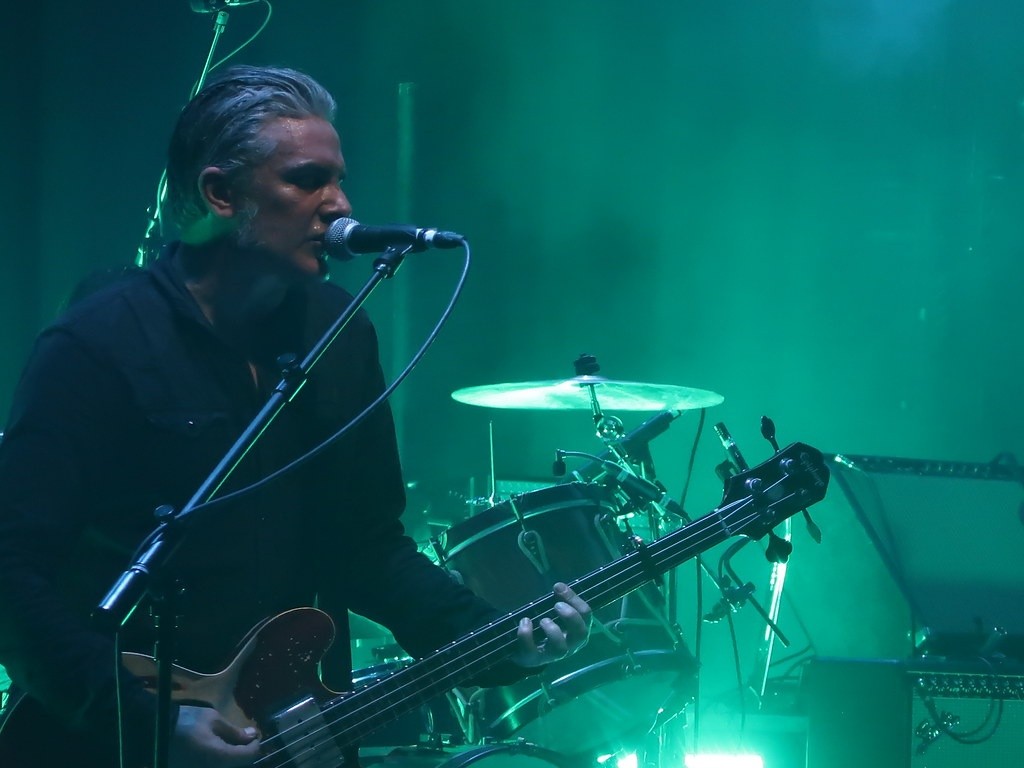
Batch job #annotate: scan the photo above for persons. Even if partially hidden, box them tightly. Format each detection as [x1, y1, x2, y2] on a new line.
[0, 65, 593, 768]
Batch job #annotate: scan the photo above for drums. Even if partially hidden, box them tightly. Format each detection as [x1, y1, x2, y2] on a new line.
[361, 736, 592, 768]
[348, 477, 701, 764]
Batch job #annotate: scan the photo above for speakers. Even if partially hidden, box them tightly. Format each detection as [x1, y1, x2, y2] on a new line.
[899, 655, 1024, 768]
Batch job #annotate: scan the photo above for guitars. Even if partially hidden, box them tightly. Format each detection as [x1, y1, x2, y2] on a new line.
[0, 412, 832, 768]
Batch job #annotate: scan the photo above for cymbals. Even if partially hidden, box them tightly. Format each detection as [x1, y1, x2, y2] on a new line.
[450, 374, 725, 413]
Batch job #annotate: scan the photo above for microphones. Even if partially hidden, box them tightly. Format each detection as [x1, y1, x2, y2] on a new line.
[190, 0, 262, 14]
[562, 410, 684, 483]
[324, 217, 465, 261]
[551, 449, 566, 478]
